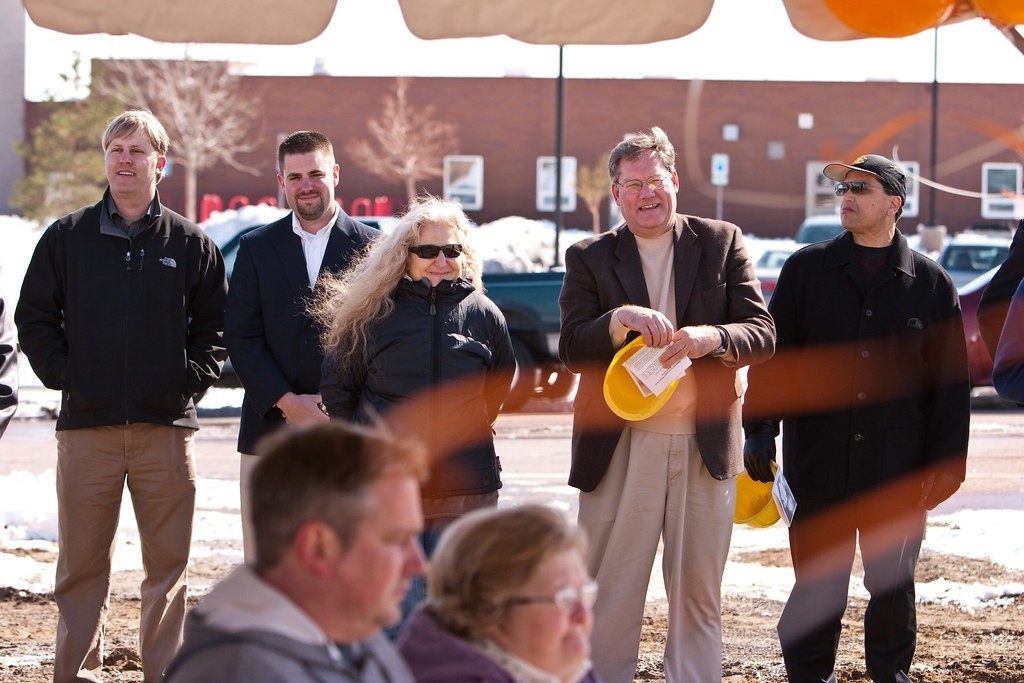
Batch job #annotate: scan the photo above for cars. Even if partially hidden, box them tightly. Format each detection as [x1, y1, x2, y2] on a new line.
[934, 231, 1015, 290]
[954, 263, 1003, 393]
[795, 212, 847, 246]
[747, 247, 806, 309]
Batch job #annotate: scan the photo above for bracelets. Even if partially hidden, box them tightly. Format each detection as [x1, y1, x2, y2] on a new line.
[714, 328, 727, 354]
[317, 402, 330, 416]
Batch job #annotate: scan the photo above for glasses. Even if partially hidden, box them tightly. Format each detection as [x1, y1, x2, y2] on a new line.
[617, 170, 669, 193]
[408, 243, 463, 259]
[835, 181, 893, 196]
[504, 582, 598, 614]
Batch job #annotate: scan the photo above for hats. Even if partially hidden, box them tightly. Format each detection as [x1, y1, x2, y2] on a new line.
[823, 154, 905, 206]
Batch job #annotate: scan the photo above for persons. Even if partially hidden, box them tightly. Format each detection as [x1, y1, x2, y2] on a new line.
[15, 109, 230, 683]
[307, 193, 518, 562]
[556, 125, 777, 682]
[229, 131, 396, 565]
[157, 422, 432, 683]
[741, 156, 971, 683]
[396, 500, 602, 683]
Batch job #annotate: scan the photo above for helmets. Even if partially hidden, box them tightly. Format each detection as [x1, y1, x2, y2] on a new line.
[733, 462, 782, 527]
[601, 332, 683, 421]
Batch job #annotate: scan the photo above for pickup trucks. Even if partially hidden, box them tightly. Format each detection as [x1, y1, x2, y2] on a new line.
[195, 214, 576, 414]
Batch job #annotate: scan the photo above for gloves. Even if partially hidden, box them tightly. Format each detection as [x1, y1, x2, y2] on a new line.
[921, 467, 962, 510]
[741, 420, 781, 483]
